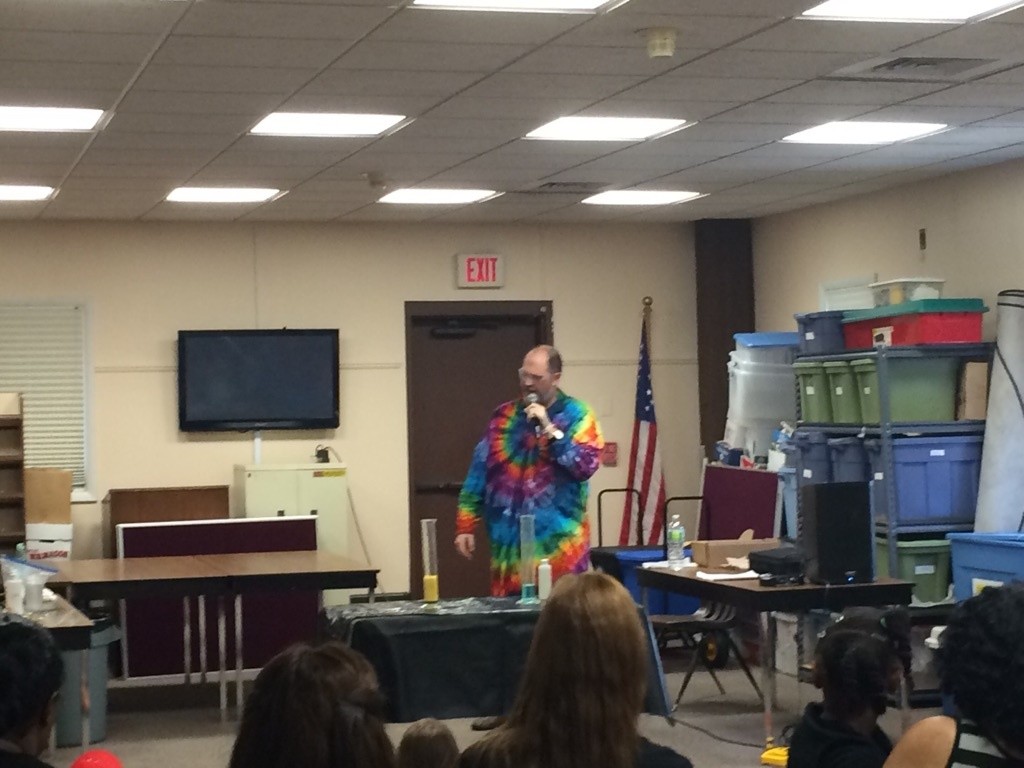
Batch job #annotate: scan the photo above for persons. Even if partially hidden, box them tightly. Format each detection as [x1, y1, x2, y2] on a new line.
[460, 573, 694, 767]
[454, 345, 603, 730]
[1, 613, 63, 768]
[787, 605, 893, 768]
[934, 581, 1024, 768]
[397, 716, 458, 767]
[227, 643, 396, 768]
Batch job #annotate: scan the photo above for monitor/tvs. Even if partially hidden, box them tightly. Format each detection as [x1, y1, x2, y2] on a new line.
[175, 327, 338, 431]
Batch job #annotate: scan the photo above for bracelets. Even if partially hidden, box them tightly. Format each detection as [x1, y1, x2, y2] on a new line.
[541, 424, 555, 434]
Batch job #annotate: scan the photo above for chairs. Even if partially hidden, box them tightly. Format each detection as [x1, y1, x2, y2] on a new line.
[880, 715, 961, 768]
[648, 595, 765, 712]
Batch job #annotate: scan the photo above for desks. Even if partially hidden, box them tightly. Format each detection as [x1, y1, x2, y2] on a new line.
[0, 590, 95, 757]
[317, 593, 671, 722]
[34, 545, 382, 712]
[642, 559, 918, 750]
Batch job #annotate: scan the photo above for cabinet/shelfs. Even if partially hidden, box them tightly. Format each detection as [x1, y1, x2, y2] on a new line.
[786, 335, 996, 710]
[232, 463, 352, 605]
[0, 391, 26, 556]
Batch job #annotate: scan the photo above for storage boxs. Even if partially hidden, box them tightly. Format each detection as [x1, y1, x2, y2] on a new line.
[25, 522, 73, 562]
[593, 276, 1024, 693]
[22, 469, 72, 524]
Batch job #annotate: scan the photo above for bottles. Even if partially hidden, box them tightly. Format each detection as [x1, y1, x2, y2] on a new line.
[667, 515, 687, 571]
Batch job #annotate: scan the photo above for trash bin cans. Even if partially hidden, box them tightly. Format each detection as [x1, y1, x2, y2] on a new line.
[46, 612, 116, 748]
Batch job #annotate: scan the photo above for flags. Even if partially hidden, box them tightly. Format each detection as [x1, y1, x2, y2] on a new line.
[618, 317, 667, 546]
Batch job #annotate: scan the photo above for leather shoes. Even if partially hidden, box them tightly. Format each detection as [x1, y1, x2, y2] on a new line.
[471, 713, 509, 729]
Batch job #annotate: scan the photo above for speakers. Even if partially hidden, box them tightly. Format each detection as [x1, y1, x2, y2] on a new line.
[801, 479, 874, 584]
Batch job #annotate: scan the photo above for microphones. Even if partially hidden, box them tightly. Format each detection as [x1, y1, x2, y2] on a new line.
[527, 393, 543, 439]
